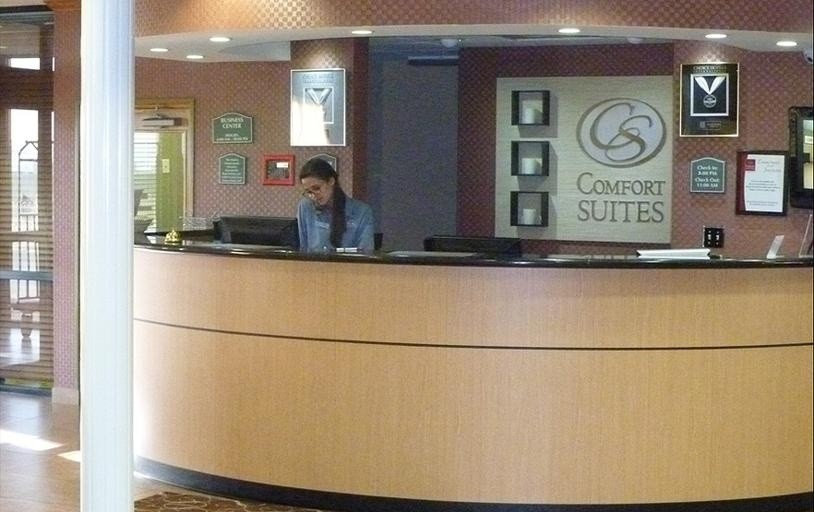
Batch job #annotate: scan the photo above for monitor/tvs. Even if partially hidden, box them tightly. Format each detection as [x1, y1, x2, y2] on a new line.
[423, 235, 522, 255]
[218, 215, 300, 248]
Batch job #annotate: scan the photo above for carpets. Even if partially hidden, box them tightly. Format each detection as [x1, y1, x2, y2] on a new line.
[137, 490, 322, 512]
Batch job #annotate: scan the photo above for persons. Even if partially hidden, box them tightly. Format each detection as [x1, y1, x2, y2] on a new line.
[294, 157, 375, 251]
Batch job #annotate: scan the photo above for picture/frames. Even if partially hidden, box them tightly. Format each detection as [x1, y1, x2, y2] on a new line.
[735, 150, 788, 216]
[289, 68, 346, 147]
[679, 62, 740, 137]
[788, 106, 813, 209]
[261, 155, 296, 185]
